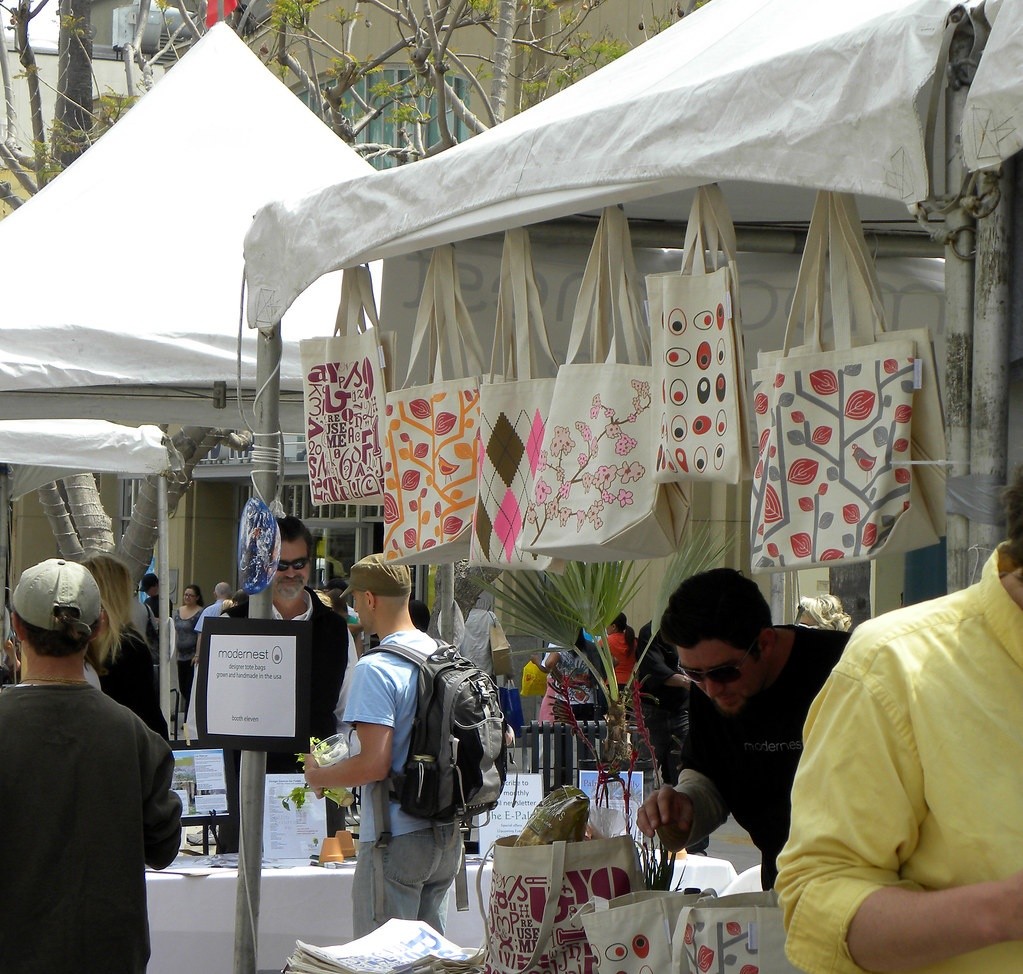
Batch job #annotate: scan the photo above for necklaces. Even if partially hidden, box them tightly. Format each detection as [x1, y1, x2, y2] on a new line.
[20, 676, 88, 685]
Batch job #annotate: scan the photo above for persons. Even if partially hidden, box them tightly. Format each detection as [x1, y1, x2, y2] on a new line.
[633, 565, 858, 892]
[307, 553, 467, 940]
[774, 478, 1023, 974]
[0, 552, 853, 857]
[218, 516, 359, 856]
[0, 556, 184, 974]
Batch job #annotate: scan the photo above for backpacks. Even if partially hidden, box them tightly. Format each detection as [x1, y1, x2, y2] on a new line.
[359, 639, 519, 823]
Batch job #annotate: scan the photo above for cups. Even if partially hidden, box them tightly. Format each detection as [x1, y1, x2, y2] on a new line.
[312, 733, 351, 768]
[334, 831, 355, 858]
[320, 837, 343, 862]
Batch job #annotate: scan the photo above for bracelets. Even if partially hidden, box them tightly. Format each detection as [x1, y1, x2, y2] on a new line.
[194, 653, 200, 658]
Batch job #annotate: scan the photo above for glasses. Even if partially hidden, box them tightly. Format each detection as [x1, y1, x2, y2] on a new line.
[676, 638, 758, 685]
[184, 593, 197, 598]
[278, 557, 309, 572]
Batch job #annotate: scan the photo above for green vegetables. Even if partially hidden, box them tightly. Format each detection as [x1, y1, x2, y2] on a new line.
[282, 738, 354, 812]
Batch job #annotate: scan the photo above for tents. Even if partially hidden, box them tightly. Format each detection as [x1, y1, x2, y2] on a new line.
[237, 0, 1023, 974]
[0, 418, 178, 740]
[0, 21, 391, 431]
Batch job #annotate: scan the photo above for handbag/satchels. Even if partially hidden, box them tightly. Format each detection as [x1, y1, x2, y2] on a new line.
[673, 889, 811, 974]
[476, 833, 645, 974]
[570, 889, 719, 974]
[520, 655, 549, 697]
[497, 679, 526, 739]
[144, 603, 159, 644]
[299, 185, 946, 577]
[487, 609, 515, 676]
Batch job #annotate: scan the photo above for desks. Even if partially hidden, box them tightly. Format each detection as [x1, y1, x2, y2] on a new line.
[145, 853, 495, 974]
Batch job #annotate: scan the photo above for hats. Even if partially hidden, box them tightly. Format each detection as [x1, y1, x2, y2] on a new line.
[139, 574, 158, 592]
[338, 554, 411, 599]
[13, 558, 101, 632]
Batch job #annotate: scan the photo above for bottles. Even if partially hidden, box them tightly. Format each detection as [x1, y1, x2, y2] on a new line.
[403, 753, 437, 817]
